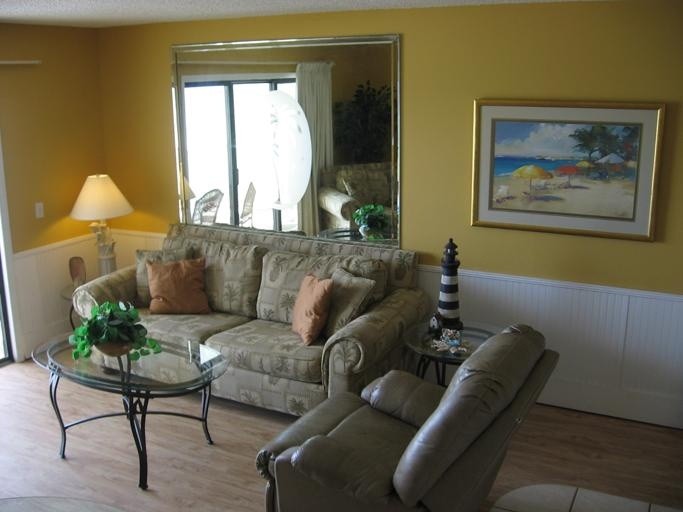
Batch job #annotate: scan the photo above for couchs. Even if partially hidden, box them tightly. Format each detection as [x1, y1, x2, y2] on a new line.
[71, 221, 430, 419]
[318, 160, 395, 230]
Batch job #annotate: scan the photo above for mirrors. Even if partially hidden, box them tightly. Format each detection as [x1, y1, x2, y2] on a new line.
[167, 31, 404, 250]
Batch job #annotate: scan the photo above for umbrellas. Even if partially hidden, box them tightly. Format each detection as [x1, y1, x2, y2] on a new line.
[512, 153, 624, 196]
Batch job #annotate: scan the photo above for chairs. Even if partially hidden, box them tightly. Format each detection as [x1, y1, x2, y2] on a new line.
[190, 188, 225, 227]
[252, 322, 561, 511]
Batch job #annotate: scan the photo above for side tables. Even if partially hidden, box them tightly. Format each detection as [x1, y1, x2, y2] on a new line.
[60, 277, 94, 332]
[403, 323, 495, 389]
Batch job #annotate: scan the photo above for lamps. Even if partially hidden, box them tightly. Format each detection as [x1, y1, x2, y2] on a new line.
[70, 173, 135, 278]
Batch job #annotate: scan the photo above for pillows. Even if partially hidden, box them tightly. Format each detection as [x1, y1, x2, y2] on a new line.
[290, 268, 375, 348]
[131, 248, 214, 314]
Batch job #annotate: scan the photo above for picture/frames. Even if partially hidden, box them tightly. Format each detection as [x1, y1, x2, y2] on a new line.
[469, 97, 667, 244]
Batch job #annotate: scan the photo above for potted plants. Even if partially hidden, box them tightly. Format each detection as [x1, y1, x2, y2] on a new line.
[69, 300, 163, 362]
[351, 204, 392, 240]
